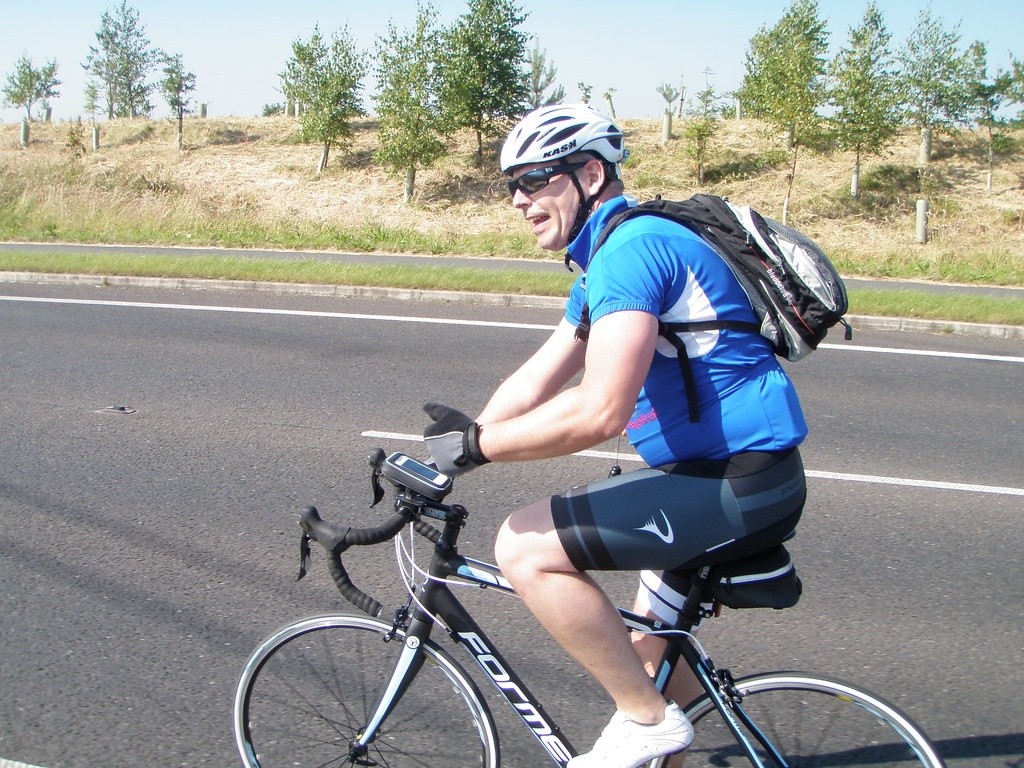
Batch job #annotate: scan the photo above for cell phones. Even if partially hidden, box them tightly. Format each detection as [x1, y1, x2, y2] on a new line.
[387, 451, 452, 491]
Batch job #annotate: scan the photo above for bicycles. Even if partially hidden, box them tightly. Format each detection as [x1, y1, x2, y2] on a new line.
[233, 444, 945, 768]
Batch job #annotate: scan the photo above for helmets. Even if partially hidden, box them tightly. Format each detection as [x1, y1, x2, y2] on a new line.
[500, 105, 626, 173]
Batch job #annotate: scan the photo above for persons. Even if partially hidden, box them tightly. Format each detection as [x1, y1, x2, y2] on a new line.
[423, 104, 811, 768]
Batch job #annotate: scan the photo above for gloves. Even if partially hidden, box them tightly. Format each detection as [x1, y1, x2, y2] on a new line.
[422, 403, 491, 475]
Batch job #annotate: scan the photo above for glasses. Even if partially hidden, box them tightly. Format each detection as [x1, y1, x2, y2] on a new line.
[507, 163, 588, 196]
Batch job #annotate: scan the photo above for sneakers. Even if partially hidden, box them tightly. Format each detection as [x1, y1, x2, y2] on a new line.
[566, 697, 694, 768]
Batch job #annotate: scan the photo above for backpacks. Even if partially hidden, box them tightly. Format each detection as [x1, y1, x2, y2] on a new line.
[577, 193, 852, 422]
